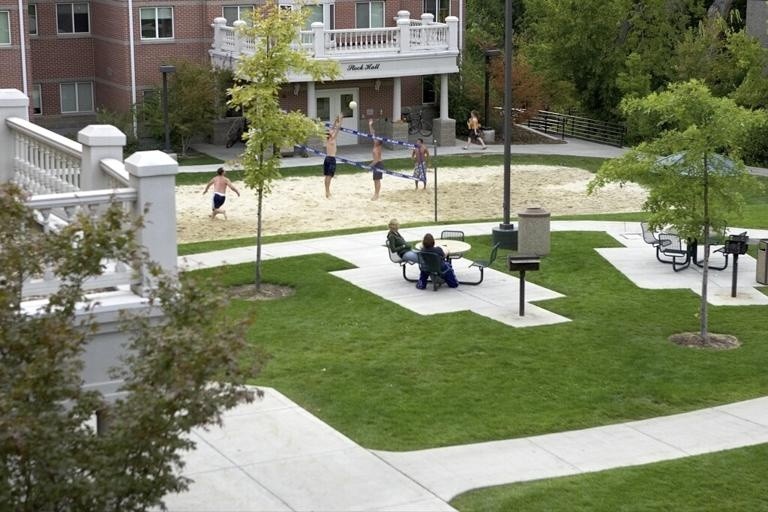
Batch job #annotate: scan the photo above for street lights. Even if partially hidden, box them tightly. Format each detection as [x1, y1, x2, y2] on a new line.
[158, 63, 178, 156]
[479, 48, 501, 131]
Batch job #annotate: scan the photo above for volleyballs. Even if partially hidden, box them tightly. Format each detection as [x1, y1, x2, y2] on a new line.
[349, 101, 357, 109]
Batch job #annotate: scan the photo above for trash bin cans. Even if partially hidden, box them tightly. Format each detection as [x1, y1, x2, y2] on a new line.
[518, 207, 551, 256]
[756, 239, 768, 284]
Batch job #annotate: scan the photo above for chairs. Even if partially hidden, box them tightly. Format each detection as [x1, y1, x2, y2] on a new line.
[385, 230, 502, 291]
[641, 219, 732, 271]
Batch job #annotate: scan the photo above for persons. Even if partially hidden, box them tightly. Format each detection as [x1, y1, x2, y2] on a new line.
[463, 111, 489, 150]
[411, 136, 430, 193]
[206, 168, 238, 220]
[367, 116, 381, 203]
[386, 218, 456, 290]
[324, 116, 344, 196]
